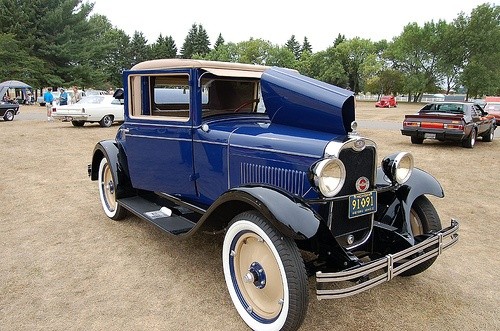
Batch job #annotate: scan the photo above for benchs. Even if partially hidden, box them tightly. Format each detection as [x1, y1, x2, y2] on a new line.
[152, 106, 253, 118]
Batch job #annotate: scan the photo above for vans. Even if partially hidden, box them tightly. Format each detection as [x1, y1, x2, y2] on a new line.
[86, 90, 108, 103]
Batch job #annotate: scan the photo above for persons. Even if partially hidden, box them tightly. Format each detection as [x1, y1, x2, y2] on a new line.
[108, 87, 115, 95]
[4, 93, 31, 106]
[71, 85, 82, 105]
[44, 87, 54, 120]
[56, 88, 67, 121]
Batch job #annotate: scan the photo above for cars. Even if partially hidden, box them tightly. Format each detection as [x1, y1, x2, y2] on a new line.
[481, 97, 500, 125]
[86, 57, 459, 331]
[374, 95, 398, 108]
[50, 95, 125, 127]
[401, 101, 497, 149]
[53, 90, 85, 107]
[0, 85, 20, 122]
[38, 92, 61, 107]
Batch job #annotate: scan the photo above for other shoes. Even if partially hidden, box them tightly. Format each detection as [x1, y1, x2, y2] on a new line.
[48, 116, 51, 121]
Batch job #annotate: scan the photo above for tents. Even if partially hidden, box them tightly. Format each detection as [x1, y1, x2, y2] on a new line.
[0, 80, 33, 104]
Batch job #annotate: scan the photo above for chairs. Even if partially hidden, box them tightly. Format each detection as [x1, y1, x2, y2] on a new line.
[430, 108, 479, 116]
